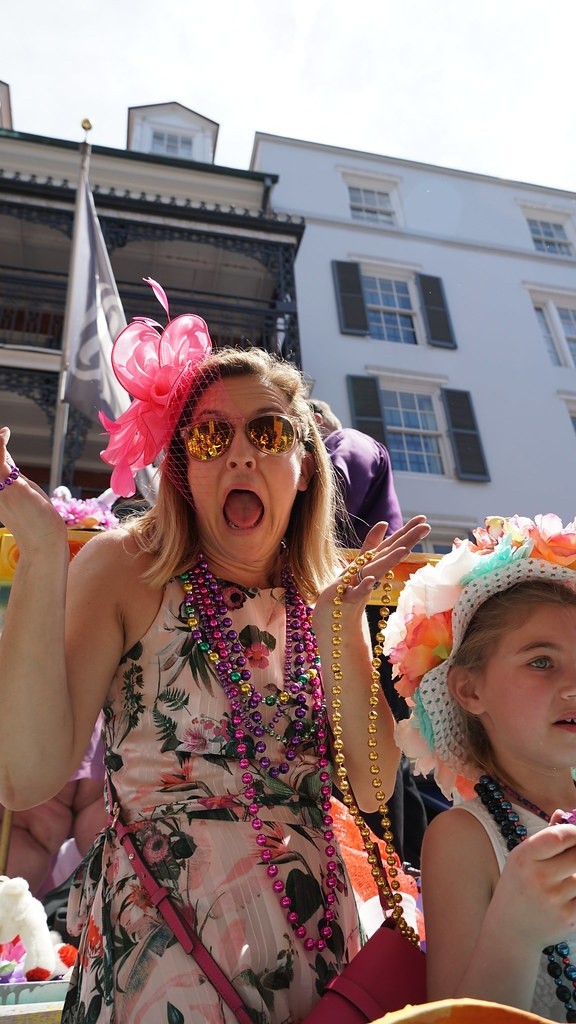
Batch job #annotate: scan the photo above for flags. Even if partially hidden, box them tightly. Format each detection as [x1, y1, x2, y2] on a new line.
[63, 183, 160, 508]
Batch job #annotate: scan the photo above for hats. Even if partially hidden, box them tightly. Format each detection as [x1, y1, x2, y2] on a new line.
[383, 512, 576, 801]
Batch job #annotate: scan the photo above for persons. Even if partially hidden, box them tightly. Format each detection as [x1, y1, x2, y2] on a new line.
[305, 397, 411, 725]
[250, 427, 292, 454]
[0, 278, 432, 1024]
[6, 710, 110, 893]
[188, 432, 229, 460]
[381, 513, 576, 1024]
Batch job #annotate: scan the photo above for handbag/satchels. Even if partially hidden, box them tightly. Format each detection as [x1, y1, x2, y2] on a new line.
[300, 917, 426, 1024]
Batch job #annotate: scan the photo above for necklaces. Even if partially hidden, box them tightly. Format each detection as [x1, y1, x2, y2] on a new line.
[473, 775, 576, 1024]
[330, 552, 420, 945]
[0, 460, 19, 491]
[179, 553, 338, 952]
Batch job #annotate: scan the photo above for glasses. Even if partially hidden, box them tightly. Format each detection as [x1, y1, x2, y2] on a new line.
[180, 415, 304, 463]
